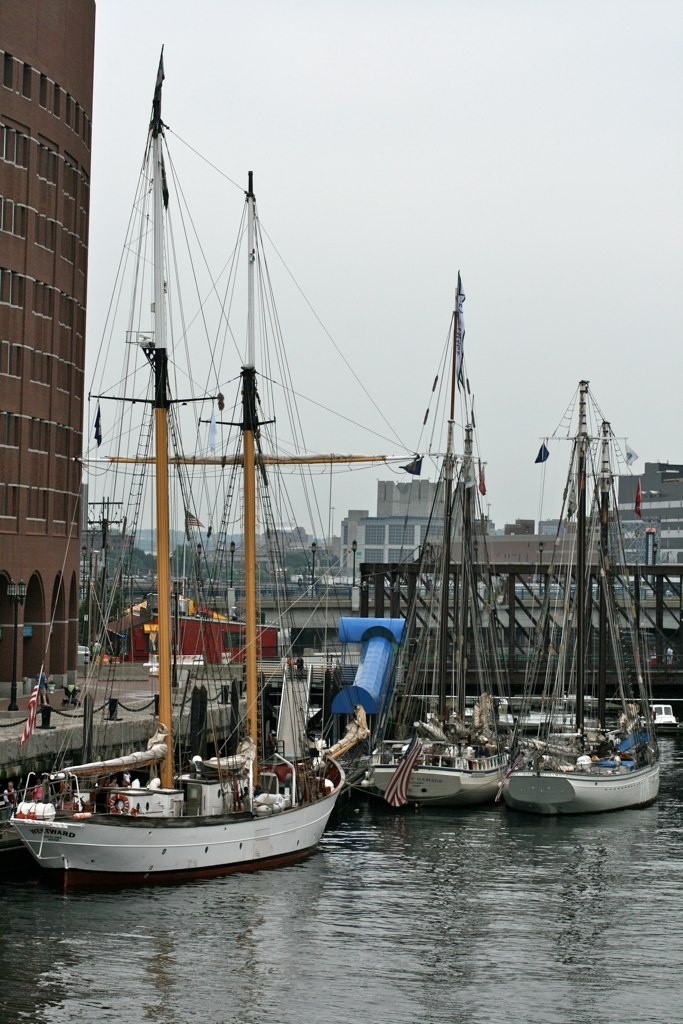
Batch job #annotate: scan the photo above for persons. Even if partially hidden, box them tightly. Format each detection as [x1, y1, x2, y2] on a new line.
[90, 641, 101, 659]
[465, 746, 476, 769]
[3, 782, 18, 808]
[613, 754, 622, 772]
[36, 668, 48, 706]
[31, 779, 43, 801]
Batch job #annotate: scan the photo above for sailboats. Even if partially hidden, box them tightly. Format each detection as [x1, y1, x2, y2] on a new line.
[343, 268, 683, 818]
[6, 45, 419, 891]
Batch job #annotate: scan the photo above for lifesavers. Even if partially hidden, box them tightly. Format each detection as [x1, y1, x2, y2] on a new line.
[110, 794, 129, 815]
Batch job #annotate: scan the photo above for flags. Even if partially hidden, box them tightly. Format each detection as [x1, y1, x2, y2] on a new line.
[534, 443, 550, 464]
[566, 478, 577, 520]
[633, 478, 642, 519]
[398, 455, 423, 475]
[479, 462, 487, 496]
[18, 684, 41, 747]
[383, 732, 423, 806]
[450, 265, 468, 395]
[624, 444, 639, 467]
[94, 405, 103, 448]
[184, 508, 206, 529]
